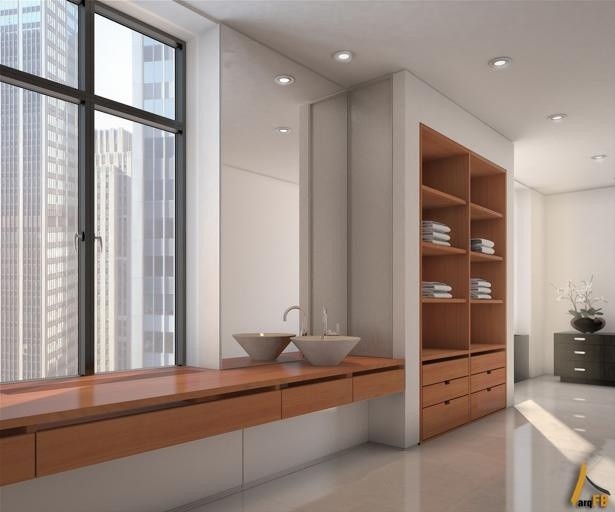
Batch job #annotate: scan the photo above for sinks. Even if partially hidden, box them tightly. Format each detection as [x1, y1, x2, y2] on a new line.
[232, 331, 296, 362]
[290, 334, 362, 366]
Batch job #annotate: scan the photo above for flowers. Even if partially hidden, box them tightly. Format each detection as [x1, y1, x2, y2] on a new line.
[554, 275, 607, 316]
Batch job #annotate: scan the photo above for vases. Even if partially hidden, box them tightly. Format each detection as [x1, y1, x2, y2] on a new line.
[571, 316, 606, 334]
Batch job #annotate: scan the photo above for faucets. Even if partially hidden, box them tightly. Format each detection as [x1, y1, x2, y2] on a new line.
[283, 305, 308, 336]
[320, 305, 340, 336]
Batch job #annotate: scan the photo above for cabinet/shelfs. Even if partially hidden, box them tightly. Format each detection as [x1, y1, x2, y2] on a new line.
[0, 354, 405, 512]
[553, 331, 615, 386]
[346, 70, 517, 447]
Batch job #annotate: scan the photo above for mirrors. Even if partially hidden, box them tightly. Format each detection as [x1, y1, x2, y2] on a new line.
[219, 24, 348, 370]
